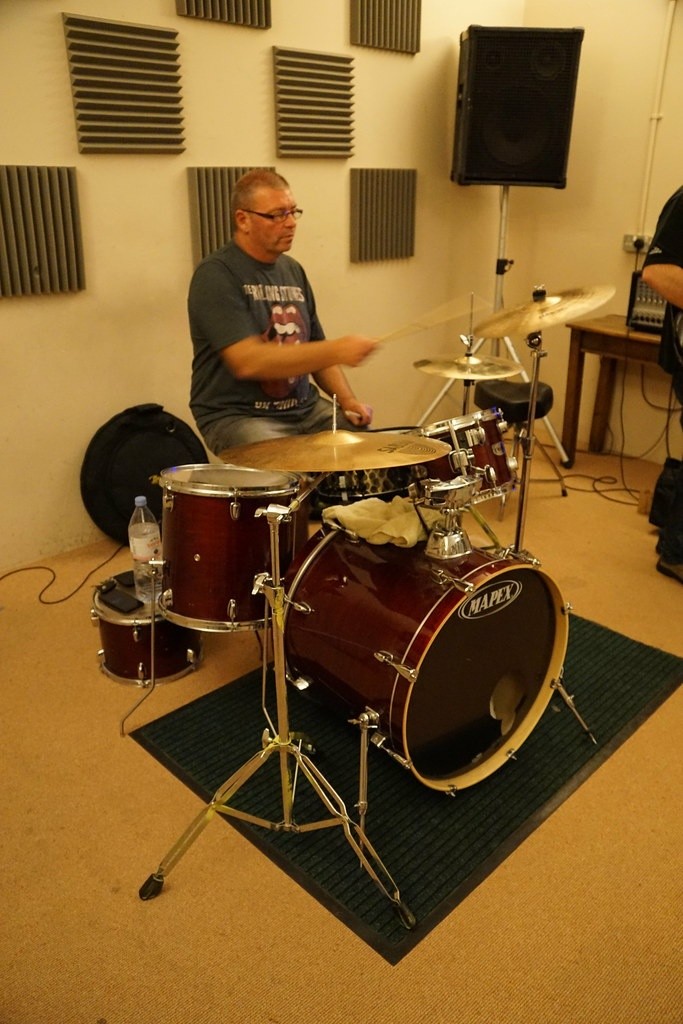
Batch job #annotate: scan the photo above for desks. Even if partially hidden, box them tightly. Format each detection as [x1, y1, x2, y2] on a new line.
[560, 313, 662, 469]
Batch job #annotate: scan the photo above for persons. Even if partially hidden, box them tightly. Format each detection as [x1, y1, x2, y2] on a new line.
[639, 184, 682, 583]
[186, 168, 384, 467]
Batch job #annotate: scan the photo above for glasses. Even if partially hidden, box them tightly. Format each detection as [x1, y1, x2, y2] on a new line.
[240, 208, 304, 222]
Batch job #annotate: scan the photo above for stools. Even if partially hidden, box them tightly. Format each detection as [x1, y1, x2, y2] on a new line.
[474, 380, 568, 497]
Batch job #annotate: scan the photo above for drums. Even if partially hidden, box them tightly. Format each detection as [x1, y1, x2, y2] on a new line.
[279, 515, 571, 791]
[147, 460, 305, 634]
[395, 405, 521, 510]
[89, 569, 201, 689]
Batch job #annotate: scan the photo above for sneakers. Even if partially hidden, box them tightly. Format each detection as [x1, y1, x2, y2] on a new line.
[656, 557, 683, 584]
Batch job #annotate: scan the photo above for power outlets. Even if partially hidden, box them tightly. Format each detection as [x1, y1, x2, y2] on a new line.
[623, 234, 652, 253]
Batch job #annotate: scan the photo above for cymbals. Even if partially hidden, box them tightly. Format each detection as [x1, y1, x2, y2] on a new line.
[412, 351, 524, 381]
[472, 282, 618, 341]
[215, 427, 455, 475]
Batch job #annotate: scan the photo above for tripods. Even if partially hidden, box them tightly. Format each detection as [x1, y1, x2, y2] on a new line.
[415, 185, 574, 469]
[139, 473, 418, 934]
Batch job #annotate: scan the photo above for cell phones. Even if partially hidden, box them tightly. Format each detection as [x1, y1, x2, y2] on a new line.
[99, 589, 144, 614]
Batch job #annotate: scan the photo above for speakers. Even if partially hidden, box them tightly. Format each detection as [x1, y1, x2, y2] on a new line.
[449, 25, 584, 189]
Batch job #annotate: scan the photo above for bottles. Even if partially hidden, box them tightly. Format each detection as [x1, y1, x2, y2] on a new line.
[128, 496, 162, 604]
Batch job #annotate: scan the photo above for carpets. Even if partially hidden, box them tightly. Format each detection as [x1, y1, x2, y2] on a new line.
[127, 611, 683, 968]
